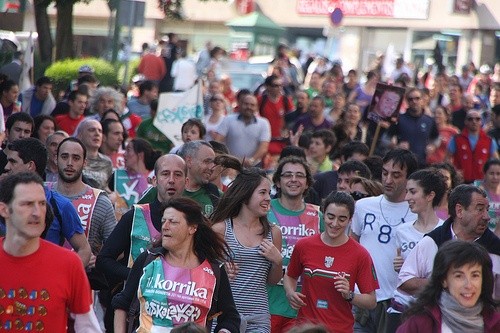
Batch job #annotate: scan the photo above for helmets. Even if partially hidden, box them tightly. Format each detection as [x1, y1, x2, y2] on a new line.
[132, 74, 148, 83]
[480, 64, 493, 74]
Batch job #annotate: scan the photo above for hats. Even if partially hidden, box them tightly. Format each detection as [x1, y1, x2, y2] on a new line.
[78, 65, 94, 74]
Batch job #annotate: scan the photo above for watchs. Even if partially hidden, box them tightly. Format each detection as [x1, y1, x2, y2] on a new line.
[346, 290, 353, 301]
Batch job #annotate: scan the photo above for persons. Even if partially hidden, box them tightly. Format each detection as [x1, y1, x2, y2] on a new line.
[0, 32, 500, 333]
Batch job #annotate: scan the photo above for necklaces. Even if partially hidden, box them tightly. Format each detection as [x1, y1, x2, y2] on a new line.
[380, 195, 410, 227]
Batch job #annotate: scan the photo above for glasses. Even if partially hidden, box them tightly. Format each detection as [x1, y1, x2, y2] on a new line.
[281, 171, 307, 179]
[350, 191, 370, 201]
[271, 84, 284, 88]
[407, 96, 422, 102]
[211, 97, 225, 103]
[467, 116, 483, 122]
